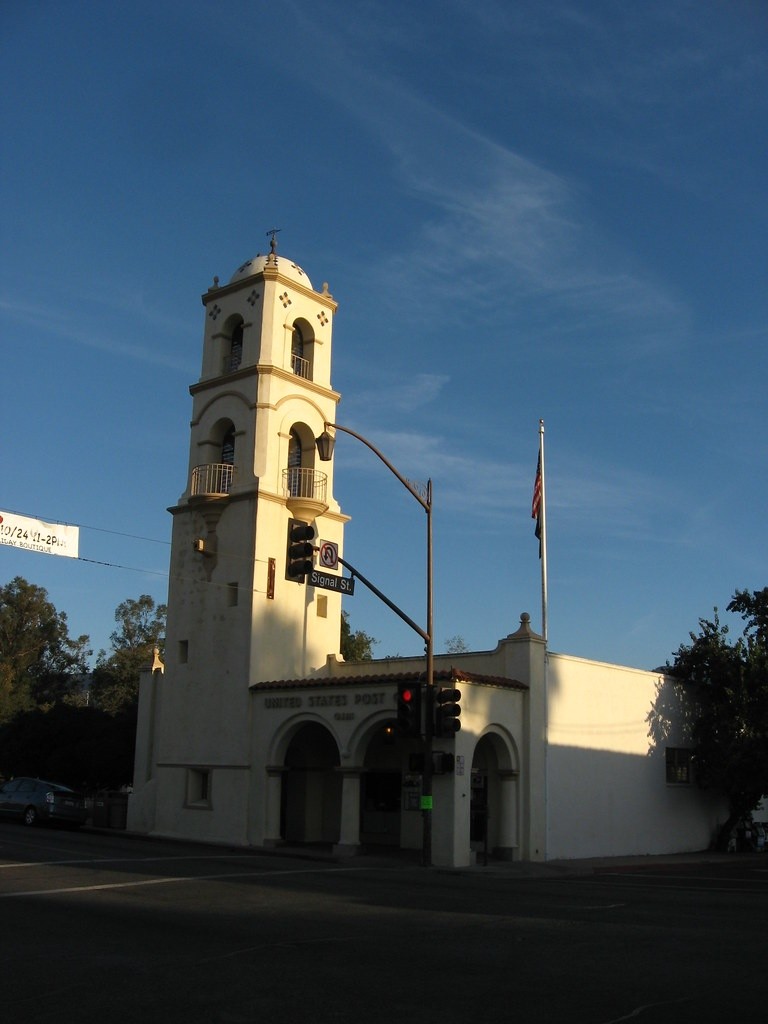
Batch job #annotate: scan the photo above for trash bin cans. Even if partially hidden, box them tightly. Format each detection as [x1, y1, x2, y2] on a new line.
[92, 790, 127, 830]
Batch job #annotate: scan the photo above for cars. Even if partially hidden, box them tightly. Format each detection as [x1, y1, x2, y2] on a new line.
[0, 776, 91, 832]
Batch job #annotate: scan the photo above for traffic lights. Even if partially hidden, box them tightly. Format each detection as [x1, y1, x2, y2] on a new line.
[393, 683, 461, 754]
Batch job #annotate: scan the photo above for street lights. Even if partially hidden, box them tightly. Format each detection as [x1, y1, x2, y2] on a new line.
[315, 419, 435, 683]
